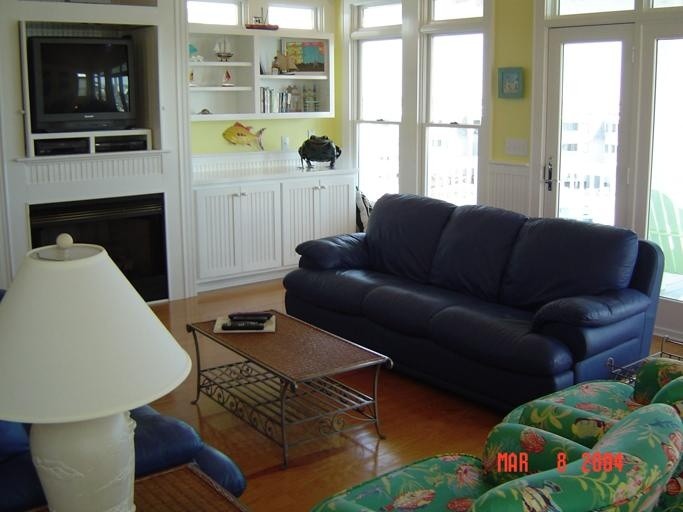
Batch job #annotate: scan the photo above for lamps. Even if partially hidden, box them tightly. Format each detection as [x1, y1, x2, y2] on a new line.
[0, 233, 194, 512]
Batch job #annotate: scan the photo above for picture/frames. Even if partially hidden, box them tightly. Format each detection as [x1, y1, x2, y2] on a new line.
[497, 67, 524, 98]
[280, 39, 329, 76]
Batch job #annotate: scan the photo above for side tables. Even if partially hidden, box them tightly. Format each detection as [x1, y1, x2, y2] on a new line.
[33, 461, 251, 512]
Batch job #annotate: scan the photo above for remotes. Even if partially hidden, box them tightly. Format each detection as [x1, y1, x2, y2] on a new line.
[228, 312, 272, 320]
[222, 321, 264, 330]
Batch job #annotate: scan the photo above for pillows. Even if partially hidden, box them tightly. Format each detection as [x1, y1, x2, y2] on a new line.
[135, 412, 205, 475]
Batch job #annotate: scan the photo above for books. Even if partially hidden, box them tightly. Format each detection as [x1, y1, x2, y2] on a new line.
[259, 85, 320, 113]
[212, 314, 277, 334]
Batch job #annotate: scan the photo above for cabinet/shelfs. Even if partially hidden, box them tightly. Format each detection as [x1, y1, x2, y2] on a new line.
[189, 22, 335, 122]
[282, 172, 356, 281]
[193, 178, 282, 294]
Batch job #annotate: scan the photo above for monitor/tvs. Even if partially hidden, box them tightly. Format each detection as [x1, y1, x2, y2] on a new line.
[32, 37, 136, 133]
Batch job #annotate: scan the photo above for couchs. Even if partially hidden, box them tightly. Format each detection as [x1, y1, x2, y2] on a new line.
[282, 193, 665, 419]
[0, 404, 248, 512]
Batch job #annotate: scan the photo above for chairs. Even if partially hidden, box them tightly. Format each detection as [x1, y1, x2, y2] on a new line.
[304, 403, 683, 512]
[502, 356, 683, 478]
